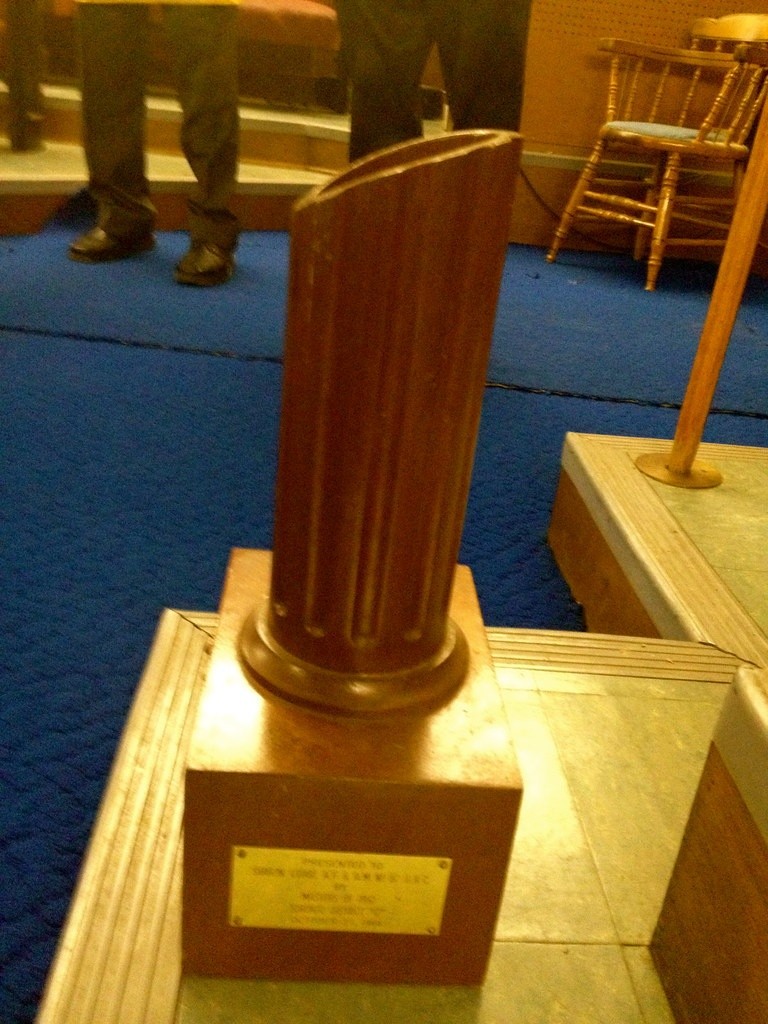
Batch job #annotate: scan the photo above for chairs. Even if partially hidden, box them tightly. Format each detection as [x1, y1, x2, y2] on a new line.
[546, 11, 768, 294]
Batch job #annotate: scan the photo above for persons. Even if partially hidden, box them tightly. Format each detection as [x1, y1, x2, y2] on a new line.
[64, 2, 242, 284]
[336, 0, 532, 164]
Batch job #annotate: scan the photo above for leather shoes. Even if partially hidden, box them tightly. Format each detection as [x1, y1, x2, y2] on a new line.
[68, 225, 157, 261]
[174, 239, 237, 286]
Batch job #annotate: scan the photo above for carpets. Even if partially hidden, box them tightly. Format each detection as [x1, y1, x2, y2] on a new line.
[0, 227, 768, 1024]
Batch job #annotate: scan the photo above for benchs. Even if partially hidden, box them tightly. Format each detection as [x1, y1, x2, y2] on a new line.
[51, 1, 340, 108]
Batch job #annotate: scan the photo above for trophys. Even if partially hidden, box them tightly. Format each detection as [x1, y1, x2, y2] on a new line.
[181, 126, 527, 991]
